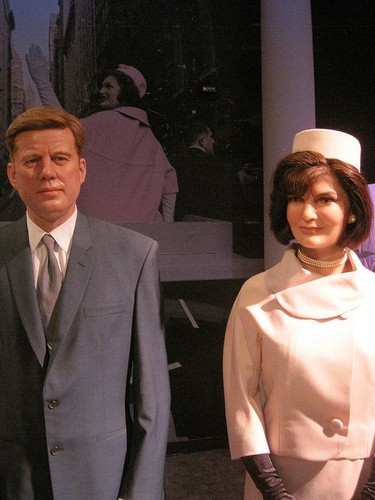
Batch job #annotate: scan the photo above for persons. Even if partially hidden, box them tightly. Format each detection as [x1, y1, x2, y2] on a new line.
[224, 129, 375, 500]
[25, 43, 177, 222]
[0, 106, 168, 500]
[175, 119, 258, 257]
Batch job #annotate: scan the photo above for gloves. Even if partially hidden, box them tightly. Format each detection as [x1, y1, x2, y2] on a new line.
[24, 44, 52, 89]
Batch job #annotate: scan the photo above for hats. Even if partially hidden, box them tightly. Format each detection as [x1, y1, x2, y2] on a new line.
[292, 129, 360, 173]
[117, 64, 148, 99]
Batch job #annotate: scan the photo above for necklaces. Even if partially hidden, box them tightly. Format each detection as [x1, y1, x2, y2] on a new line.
[297, 250, 348, 268]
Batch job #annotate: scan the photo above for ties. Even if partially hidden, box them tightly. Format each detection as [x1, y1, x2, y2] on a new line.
[38, 233, 62, 333]
